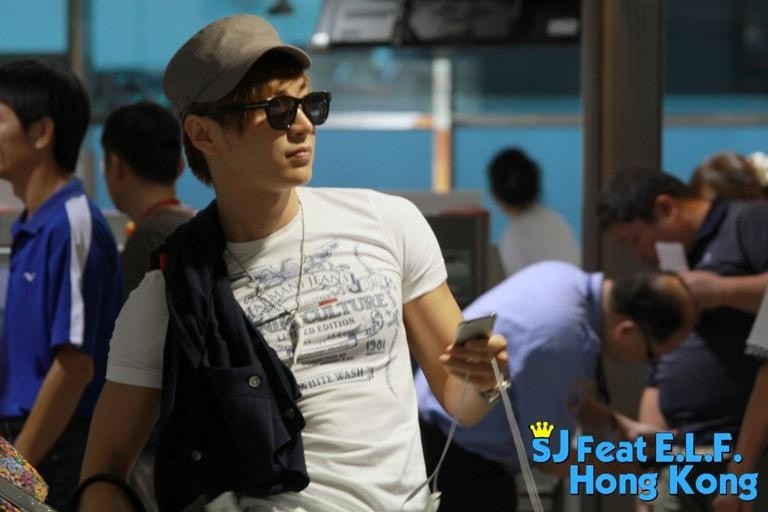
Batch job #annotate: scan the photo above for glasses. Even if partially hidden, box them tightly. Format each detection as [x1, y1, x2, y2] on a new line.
[219, 88, 333, 133]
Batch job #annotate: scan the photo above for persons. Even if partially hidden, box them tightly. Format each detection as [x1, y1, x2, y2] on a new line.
[595, 166, 768, 512]
[489, 148, 582, 278]
[100, 98, 197, 304]
[414, 260, 695, 512]
[713, 286, 768, 511]
[0, 58, 122, 510]
[78, 14, 512, 512]
[688, 150, 768, 203]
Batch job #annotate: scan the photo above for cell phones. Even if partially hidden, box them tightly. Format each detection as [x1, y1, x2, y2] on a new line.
[454, 312, 497, 346]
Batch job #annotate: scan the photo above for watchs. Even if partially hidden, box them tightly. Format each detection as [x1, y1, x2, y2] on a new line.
[478, 377, 513, 405]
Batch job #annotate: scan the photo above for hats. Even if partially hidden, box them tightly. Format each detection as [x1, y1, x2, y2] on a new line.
[162, 12, 314, 116]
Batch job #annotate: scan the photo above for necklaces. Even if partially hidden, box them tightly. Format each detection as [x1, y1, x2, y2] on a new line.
[133, 198, 181, 225]
[224, 197, 307, 367]
